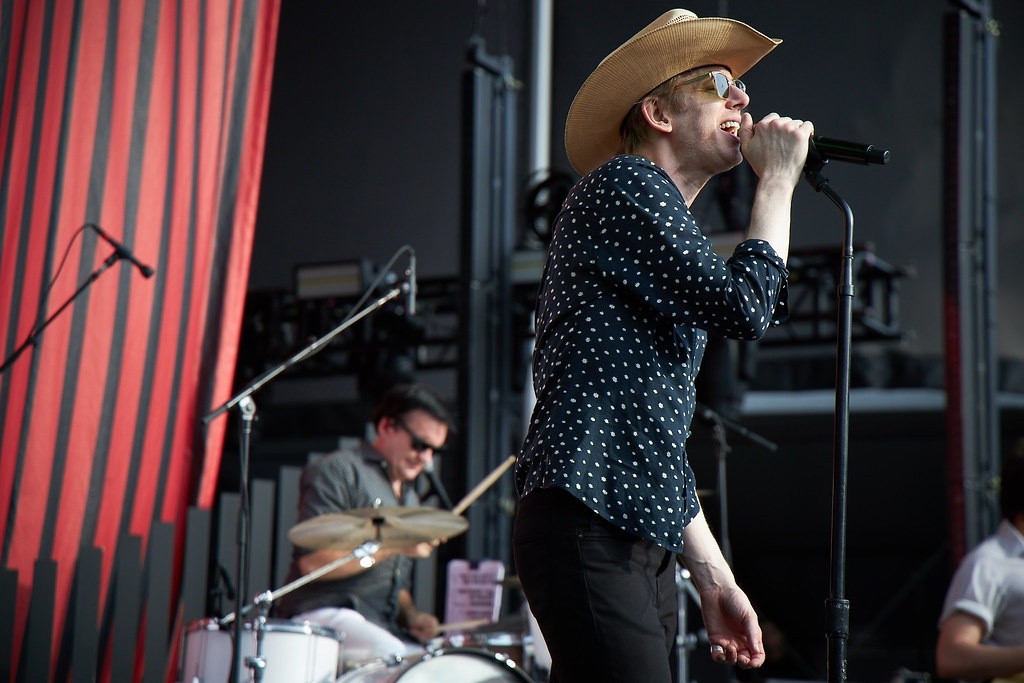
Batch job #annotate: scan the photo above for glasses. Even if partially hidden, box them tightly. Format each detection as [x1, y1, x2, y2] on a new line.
[400, 421, 447, 457]
[677, 72, 747, 100]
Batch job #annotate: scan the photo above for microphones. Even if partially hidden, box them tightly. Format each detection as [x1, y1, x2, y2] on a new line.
[402, 254, 416, 318]
[219, 559, 238, 602]
[747, 123, 890, 168]
[92, 223, 156, 278]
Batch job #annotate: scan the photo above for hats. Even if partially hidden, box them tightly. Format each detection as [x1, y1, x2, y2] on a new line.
[564, 8, 783, 176]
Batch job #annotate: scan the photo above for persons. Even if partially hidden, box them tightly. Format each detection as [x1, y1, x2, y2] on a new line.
[937, 488, 1024, 683]
[509, 9, 815, 683]
[279, 385, 451, 668]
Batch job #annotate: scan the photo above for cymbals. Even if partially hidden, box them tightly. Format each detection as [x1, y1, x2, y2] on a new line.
[445, 607, 528, 637]
[286, 504, 471, 553]
[494, 574, 522, 586]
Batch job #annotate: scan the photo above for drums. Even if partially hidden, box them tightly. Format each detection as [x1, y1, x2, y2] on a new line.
[180, 615, 343, 683]
[334, 646, 535, 683]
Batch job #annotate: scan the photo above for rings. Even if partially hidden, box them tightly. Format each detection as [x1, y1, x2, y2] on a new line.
[710, 644, 723, 653]
